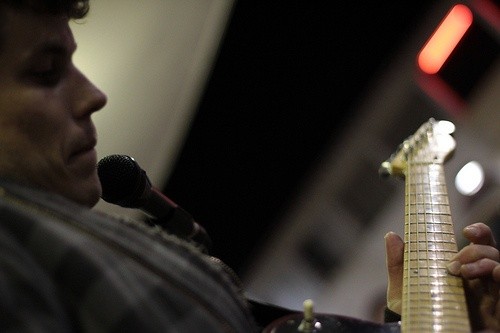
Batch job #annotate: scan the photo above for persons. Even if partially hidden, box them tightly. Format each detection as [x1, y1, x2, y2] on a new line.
[1, 0, 499, 333]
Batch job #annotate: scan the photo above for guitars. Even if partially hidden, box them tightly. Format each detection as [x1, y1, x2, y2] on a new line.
[259, 115, 473, 333]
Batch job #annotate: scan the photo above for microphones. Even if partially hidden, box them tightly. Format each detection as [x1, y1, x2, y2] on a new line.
[98, 154, 213, 253]
